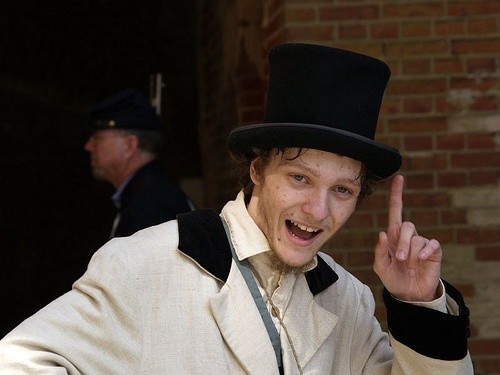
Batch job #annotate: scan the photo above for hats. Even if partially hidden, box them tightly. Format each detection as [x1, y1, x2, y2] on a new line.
[69, 94, 163, 131]
[226, 42, 402, 180]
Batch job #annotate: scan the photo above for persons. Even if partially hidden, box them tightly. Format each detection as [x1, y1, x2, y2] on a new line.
[83, 87, 198, 240]
[1, 41, 478, 375]
